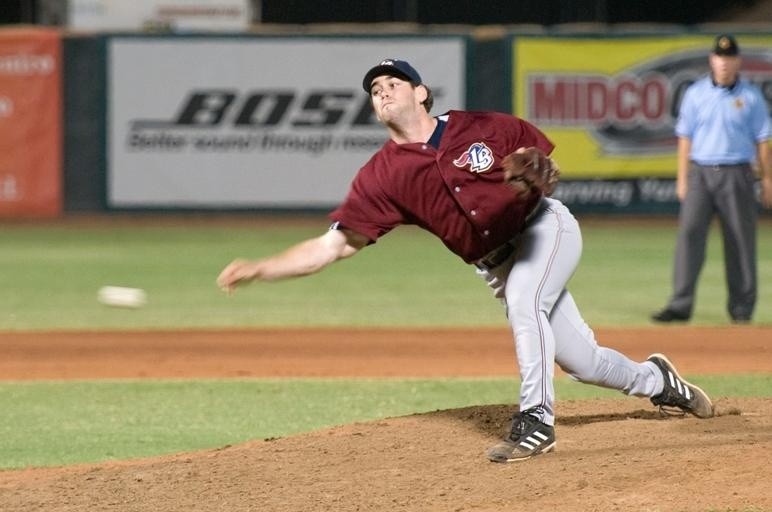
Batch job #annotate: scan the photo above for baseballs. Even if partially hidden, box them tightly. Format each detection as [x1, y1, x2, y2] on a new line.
[99, 285, 144, 308]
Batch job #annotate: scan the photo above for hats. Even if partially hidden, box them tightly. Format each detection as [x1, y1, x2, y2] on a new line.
[362, 57, 431, 109]
[710, 36, 740, 56]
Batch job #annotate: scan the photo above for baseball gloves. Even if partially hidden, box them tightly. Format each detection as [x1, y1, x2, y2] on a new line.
[501, 146, 560, 199]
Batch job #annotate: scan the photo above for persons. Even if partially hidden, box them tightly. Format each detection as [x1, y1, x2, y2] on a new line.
[653, 32, 772, 323]
[217, 58, 714, 463]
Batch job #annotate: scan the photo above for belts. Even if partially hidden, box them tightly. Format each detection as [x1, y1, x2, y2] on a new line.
[473, 199, 551, 274]
[690, 161, 748, 170]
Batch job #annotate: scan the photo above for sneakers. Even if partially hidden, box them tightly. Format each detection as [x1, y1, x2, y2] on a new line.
[647, 353, 714, 419]
[730, 306, 751, 323]
[488, 406, 556, 461]
[652, 307, 694, 322]
